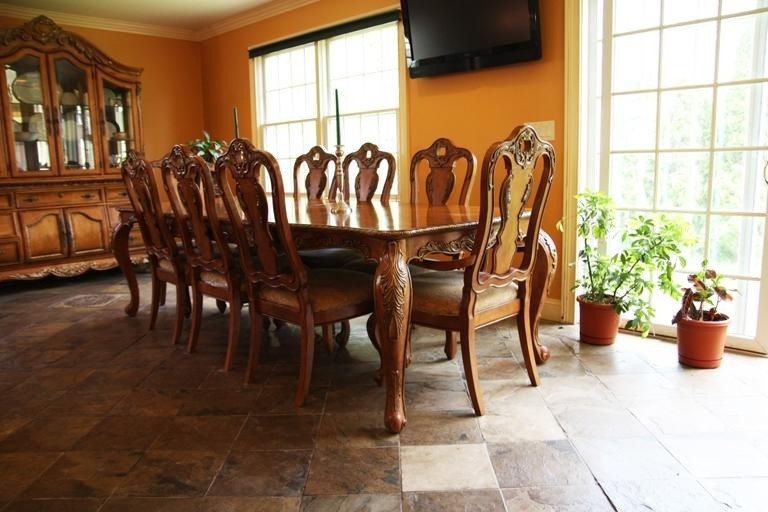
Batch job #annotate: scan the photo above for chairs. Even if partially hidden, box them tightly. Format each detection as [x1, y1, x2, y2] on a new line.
[369, 124, 561, 414]
[111, 147, 240, 347]
[160, 142, 338, 375]
[295, 145, 395, 205]
[407, 137, 478, 358]
[214, 138, 394, 409]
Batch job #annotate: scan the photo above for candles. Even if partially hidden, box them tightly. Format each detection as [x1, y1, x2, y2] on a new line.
[335, 87, 341, 146]
[232, 106, 240, 140]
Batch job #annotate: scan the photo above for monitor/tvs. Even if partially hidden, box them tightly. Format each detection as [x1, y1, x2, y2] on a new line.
[401, 0, 541, 79]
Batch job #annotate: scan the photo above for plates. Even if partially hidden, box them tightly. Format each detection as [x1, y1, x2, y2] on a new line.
[102, 88, 118, 106]
[59, 121, 84, 141]
[10, 71, 43, 104]
[61, 91, 79, 106]
[103, 119, 120, 141]
[11, 120, 22, 131]
[28, 111, 48, 143]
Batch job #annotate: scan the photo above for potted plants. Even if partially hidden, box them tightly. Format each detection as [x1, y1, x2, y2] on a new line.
[664, 260, 741, 371]
[551, 178, 701, 350]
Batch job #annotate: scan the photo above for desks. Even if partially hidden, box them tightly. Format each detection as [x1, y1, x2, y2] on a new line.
[109, 191, 553, 433]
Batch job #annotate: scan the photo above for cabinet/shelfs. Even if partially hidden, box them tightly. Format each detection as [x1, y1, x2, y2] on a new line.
[0, 12, 153, 292]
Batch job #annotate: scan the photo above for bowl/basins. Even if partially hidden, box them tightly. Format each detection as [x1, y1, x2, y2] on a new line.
[13, 131, 41, 142]
[113, 129, 128, 140]
